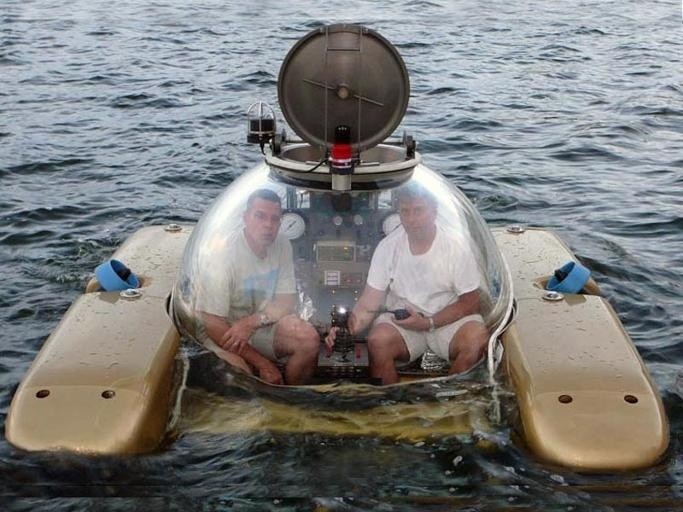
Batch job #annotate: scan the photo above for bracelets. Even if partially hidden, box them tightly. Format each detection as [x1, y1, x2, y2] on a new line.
[260, 311, 269, 326]
[429, 317, 435, 333]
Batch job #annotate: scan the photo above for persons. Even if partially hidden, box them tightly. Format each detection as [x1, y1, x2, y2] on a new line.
[324, 182, 492, 386]
[194, 188, 321, 386]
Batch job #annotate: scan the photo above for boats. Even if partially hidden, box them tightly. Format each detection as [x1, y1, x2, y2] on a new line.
[3, 23, 673, 477]
